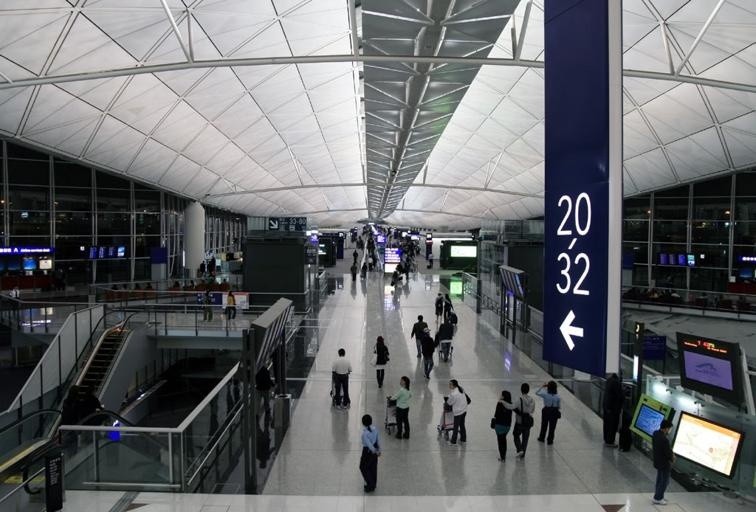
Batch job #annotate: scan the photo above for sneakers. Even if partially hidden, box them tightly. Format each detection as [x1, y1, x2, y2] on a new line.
[447, 439, 467, 446]
[403, 433, 410, 440]
[335, 404, 348, 411]
[395, 433, 403, 440]
[537, 437, 554, 445]
[497, 451, 525, 463]
[652, 497, 667, 505]
[377, 383, 383, 388]
[604, 443, 618, 448]
[364, 484, 375, 493]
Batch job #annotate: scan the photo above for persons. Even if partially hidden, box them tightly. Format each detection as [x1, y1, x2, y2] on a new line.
[387, 375, 411, 440]
[598, 442, 628, 511]
[254, 410, 276, 469]
[445, 441, 467, 491]
[492, 389, 534, 464]
[537, 438, 558, 509]
[199, 259, 206, 278]
[328, 405, 353, 465]
[485, 457, 510, 512]
[208, 260, 216, 280]
[350, 224, 433, 287]
[80, 385, 107, 445]
[651, 419, 678, 505]
[211, 254, 216, 266]
[108, 278, 229, 291]
[370, 334, 390, 389]
[498, 382, 535, 458]
[8, 283, 20, 300]
[409, 292, 459, 380]
[534, 379, 562, 445]
[331, 348, 352, 410]
[442, 379, 468, 448]
[350, 264, 433, 311]
[358, 414, 383, 493]
[253, 361, 275, 418]
[57, 384, 82, 457]
[412, 353, 453, 431]
[601, 372, 626, 448]
[507, 456, 530, 510]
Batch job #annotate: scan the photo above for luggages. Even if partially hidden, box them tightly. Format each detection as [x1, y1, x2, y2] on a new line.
[450, 312, 458, 326]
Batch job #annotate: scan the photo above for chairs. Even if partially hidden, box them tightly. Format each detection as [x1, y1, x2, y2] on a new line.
[169, 283, 229, 291]
[104, 289, 155, 301]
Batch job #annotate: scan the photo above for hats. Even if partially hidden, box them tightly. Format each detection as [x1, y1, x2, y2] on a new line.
[424, 328, 431, 333]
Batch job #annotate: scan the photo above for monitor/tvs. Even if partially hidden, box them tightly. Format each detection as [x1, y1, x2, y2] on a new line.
[668, 411, 743, 478]
[629, 393, 674, 444]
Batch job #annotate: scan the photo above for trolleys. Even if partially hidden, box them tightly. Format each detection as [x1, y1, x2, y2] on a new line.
[328, 369, 351, 408]
[438, 340, 453, 360]
[435, 403, 454, 442]
[384, 395, 398, 436]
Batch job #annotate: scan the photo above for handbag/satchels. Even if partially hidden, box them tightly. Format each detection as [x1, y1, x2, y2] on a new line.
[369, 353, 378, 367]
[465, 393, 472, 404]
[491, 417, 495, 429]
[520, 413, 534, 429]
[557, 410, 562, 419]
